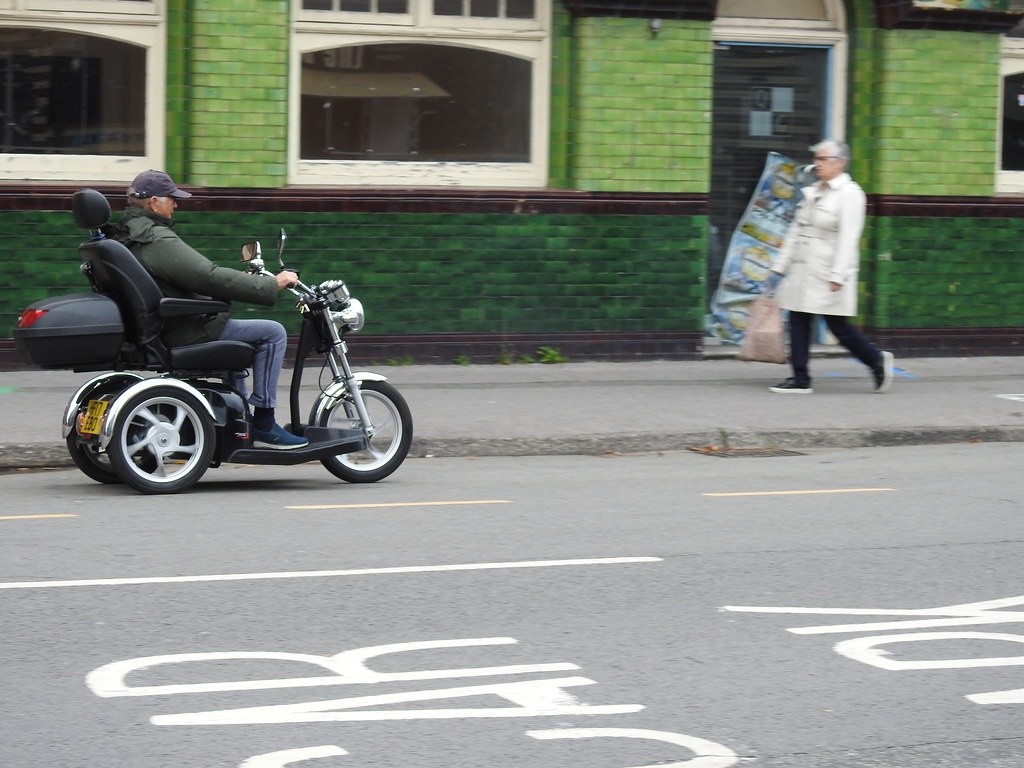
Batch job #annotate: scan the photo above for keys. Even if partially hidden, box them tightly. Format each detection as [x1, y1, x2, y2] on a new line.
[297, 297, 310, 314]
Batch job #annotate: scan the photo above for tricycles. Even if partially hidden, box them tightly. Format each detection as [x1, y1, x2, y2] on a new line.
[13, 185, 416, 496]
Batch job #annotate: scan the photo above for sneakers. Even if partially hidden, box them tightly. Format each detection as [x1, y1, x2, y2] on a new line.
[872, 351, 894, 392]
[251, 423, 309, 450]
[768, 377, 813, 393]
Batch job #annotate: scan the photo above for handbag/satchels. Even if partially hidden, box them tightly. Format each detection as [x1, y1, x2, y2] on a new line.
[740, 294, 787, 364]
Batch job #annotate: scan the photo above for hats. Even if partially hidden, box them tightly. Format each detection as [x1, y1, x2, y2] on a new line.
[128, 169, 192, 198]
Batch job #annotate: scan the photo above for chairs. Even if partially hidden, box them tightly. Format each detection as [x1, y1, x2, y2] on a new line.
[71, 188, 255, 384]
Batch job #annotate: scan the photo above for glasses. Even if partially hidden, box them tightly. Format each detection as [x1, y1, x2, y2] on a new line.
[811, 156, 837, 162]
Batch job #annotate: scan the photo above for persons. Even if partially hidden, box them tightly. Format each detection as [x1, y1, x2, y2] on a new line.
[101, 169, 308, 450]
[770, 140, 893, 395]
